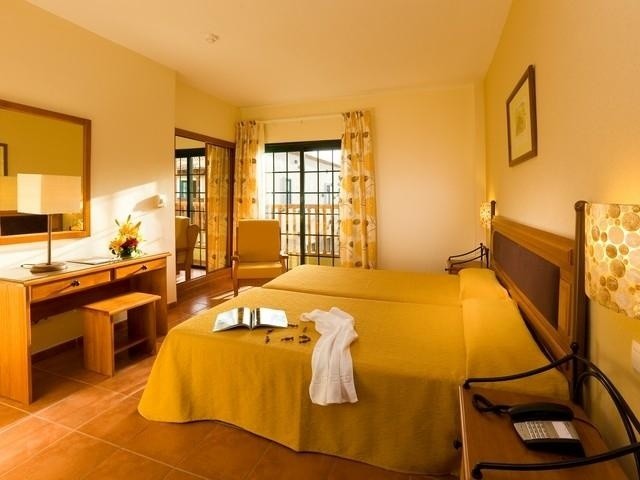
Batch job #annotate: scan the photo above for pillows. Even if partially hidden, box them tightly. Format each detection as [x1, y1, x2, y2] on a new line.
[459, 295, 573, 403]
[457, 266, 511, 297]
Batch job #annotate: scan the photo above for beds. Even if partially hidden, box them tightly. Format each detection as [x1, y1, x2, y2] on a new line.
[136, 197, 593, 480]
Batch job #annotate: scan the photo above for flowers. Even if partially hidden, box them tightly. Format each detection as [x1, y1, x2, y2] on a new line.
[103, 212, 145, 256]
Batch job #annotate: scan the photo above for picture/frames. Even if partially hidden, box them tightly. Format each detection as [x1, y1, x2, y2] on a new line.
[501, 62, 540, 167]
[0, 141, 10, 176]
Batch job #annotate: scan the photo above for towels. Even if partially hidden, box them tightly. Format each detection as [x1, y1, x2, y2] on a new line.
[302, 304, 362, 411]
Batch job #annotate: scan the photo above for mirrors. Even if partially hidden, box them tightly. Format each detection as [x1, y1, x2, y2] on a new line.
[172, 126, 236, 297]
[1, 92, 96, 246]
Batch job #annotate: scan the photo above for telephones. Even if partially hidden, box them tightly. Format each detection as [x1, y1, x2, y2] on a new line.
[508, 403, 585, 458]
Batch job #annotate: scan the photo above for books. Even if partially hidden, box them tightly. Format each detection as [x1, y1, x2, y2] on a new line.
[69, 255, 117, 264]
[212, 307, 289, 332]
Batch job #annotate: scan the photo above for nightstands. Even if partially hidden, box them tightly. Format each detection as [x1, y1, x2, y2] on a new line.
[444, 242, 491, 274]
[456, 337, 640, 479]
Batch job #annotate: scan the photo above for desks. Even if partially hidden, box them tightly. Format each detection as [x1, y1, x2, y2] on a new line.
[1, 246, 172, 408]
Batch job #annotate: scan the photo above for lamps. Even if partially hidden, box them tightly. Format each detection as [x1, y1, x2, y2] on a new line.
[581, 201, 640, 320]
[14, 170, 84, 273]
[479, 199, 497, 246]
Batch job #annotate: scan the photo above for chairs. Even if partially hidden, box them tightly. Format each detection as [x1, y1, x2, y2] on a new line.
[173, 211, 200, 280]
[230, 217, 292, 297]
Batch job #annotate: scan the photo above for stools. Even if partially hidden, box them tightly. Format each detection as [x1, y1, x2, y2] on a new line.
[81, 292, 161, 379]
